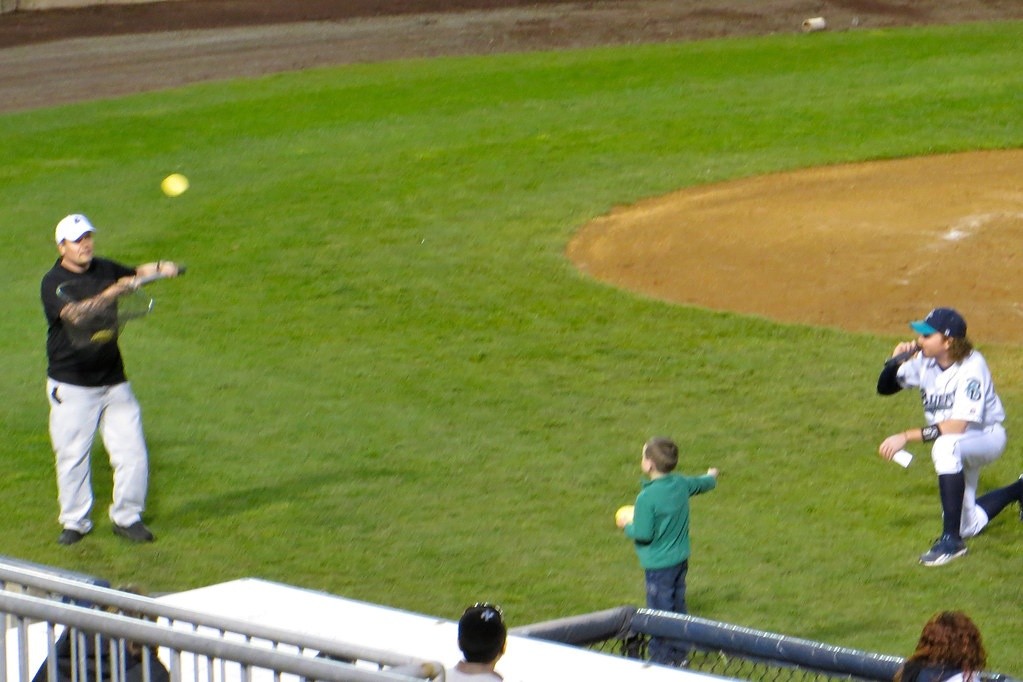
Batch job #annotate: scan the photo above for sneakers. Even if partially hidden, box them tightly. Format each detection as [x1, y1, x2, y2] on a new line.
[112, 523, 152, 543]
[57, 529, 82, 546]
[919, 533, 969, 567]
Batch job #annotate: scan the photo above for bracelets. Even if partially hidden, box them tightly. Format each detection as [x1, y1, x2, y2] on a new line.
[157, 263, 160, 271]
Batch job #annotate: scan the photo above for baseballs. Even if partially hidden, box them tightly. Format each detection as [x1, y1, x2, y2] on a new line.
[161, 173, 190, 198]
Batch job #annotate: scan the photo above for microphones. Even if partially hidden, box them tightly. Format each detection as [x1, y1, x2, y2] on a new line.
[884, 345, 922, 368]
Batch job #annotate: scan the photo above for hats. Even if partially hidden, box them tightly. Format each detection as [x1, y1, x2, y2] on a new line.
[459, 603, 505, 651]
[910, 309, 966, 341]
[55, 214, 95, 244]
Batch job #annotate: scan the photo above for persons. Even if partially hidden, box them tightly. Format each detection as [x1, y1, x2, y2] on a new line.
[42, 214, 178, 543]
[877, 308, 1022, 566]
[616, 437, 718, 670]
[892, 611, 985, 682]
[445, 604, 507, 682]
[31, 586, 169, 682]
